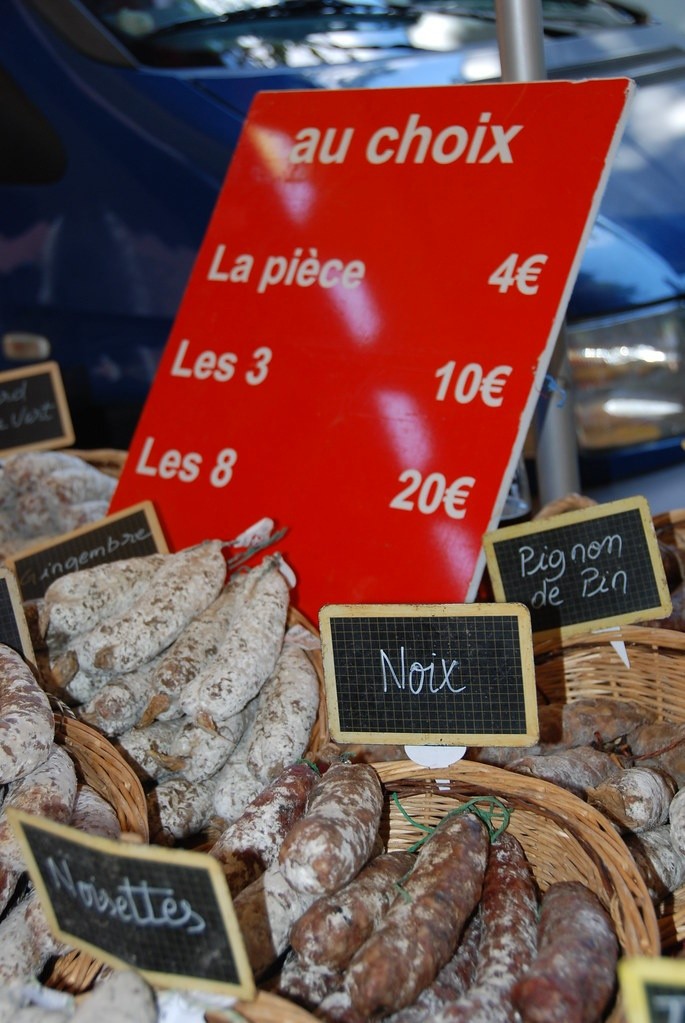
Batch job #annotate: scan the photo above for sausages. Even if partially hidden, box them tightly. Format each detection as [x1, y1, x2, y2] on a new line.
[0, 449, 685, 1023]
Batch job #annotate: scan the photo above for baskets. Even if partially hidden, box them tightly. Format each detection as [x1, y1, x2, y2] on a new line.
[283, 603, 332, 758]
[152, 983, 319, 1023]
[534, 493, 685, 626]
[39, 709, 148, 995]
[188, 759, 661, 1023]
[53, 449, 130, 481]
[347, 625, 685, 946]
